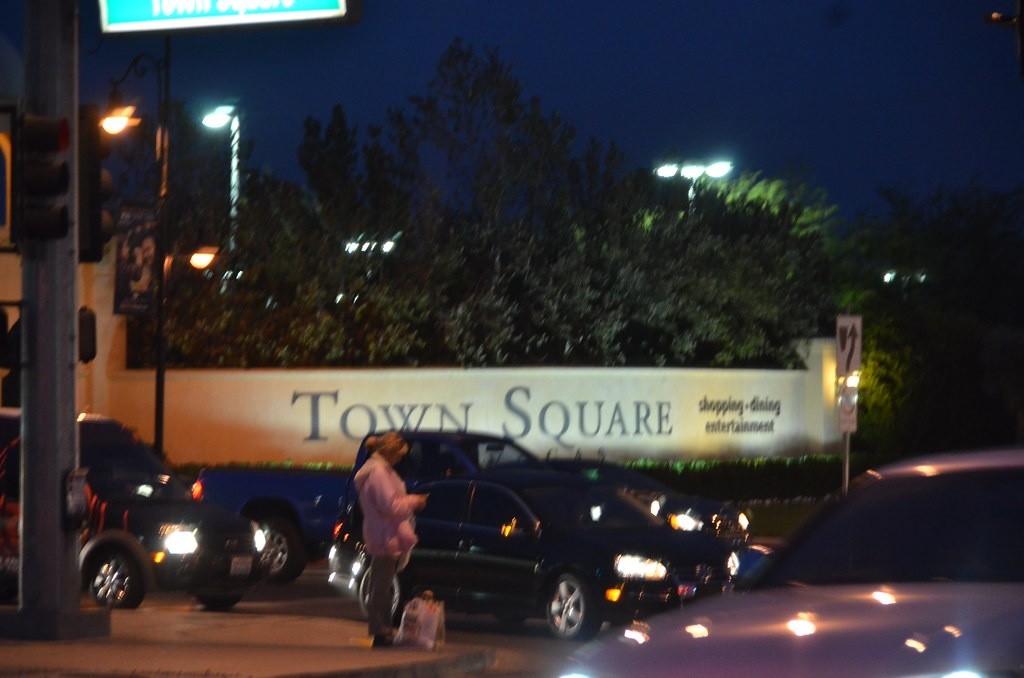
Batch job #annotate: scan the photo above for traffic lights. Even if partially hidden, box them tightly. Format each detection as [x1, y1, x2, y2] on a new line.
[8, 105, 70, 247]
[76, 103, 119, 264]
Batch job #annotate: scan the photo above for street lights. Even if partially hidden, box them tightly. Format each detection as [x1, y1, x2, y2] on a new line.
[202, 100, 244, 366]
[96, 30, 171, 460]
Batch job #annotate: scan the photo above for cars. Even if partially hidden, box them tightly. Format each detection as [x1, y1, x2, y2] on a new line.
[322, 458, 775, 646]
[0, 415, 257, 613]
[552, 449, 1024, 678]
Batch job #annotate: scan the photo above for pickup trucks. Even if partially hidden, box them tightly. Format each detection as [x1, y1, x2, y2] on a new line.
[189, 426, 540, 584]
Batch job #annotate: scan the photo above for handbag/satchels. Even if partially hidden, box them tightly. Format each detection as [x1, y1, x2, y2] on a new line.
[393, 597, 446, 652]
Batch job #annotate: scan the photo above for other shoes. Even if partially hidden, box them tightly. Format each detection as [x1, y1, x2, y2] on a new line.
[371, 634, 397, 647]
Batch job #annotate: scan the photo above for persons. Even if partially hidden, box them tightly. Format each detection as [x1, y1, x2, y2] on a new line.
[354, 430, 428, 647]
